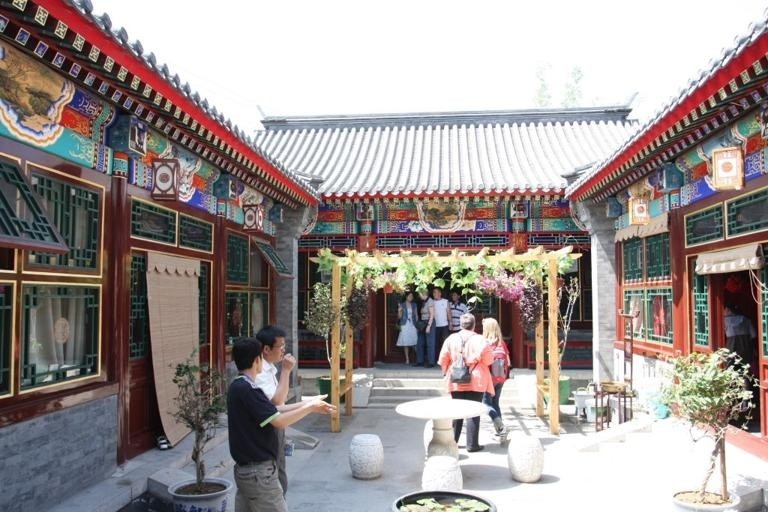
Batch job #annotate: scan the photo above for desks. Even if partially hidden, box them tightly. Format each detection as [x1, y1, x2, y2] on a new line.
[392, 395, 490, 463]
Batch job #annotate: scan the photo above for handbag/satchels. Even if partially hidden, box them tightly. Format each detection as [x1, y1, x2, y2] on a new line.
[395, 320, 402, 331]
[416, 319, 428, 329]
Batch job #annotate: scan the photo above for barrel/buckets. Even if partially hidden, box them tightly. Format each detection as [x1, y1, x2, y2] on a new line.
[584, 398, 608, 424]
[571, 387, 595, 415]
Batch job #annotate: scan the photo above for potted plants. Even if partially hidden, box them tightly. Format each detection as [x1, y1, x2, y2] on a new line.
[162, 339, 235, 512]
[299, 280, 373, 406]
[662, 346, 761, 512]
[517, 272, 583, 407]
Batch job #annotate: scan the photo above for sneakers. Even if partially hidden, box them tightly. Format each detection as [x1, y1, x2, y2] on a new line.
[500, 426, 510, 445]
[467, 445, 484, 451]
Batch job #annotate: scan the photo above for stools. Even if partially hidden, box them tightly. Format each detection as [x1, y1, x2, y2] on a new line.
[349, 433, 386, 480]
[422, 420, 435, 460]
[419, 454, 465, 492]
[506, 433, 545, 484]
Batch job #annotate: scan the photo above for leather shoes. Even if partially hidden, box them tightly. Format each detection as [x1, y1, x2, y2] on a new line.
[412, 362, 424, 367]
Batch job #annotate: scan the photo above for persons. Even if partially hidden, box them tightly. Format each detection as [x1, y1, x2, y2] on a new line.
[479, 317, 514, 434]
[394, 288, 470, 368]
[227, 338, 338, 512]
[436, 312, 494, 454]
[720, 300, 756, 431]
[249, 326, 326, 501]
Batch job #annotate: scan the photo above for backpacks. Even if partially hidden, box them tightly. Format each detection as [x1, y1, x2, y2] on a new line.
[489, 337, 509, 384]
[450, 333, 478, 383]
